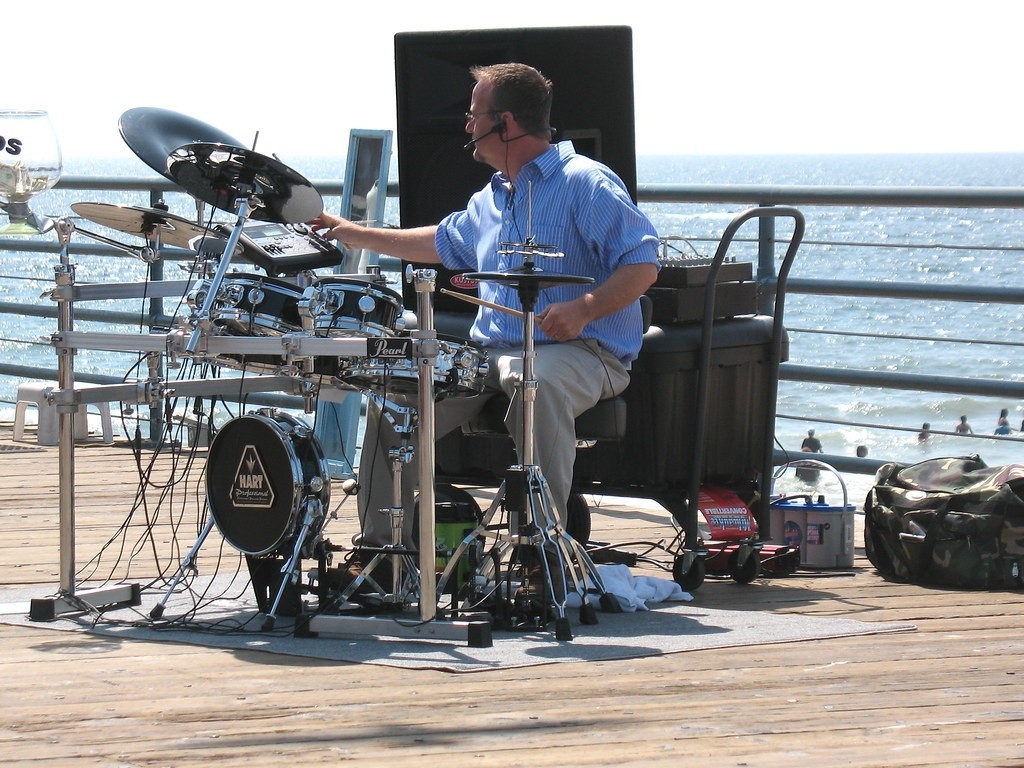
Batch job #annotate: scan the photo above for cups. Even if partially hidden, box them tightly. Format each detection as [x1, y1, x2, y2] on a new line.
[0, 111, 63, 202]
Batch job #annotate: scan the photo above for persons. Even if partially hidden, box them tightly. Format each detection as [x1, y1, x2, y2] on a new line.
[306, 63, 663, 619]
[857, 445, 868, 457]
[998, 408, 1010, 425]
[796, 447, 819, 481]
[956, 415, 972, 434]
[918, 422, 931, 438]
[994, 421, 1013, 435]
[801, 429, 824, 452]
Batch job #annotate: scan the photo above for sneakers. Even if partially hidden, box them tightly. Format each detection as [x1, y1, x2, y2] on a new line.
[306, 547, 393, 593]
[515, 565, 564, 605]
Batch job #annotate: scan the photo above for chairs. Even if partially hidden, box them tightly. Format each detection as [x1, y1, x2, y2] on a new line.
[432, 294, 651, 578]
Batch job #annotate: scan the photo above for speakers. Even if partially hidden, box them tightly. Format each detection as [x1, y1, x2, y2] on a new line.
[394, 23, 638, 314]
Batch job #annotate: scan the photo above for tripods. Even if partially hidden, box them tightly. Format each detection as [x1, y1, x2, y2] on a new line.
[316, 282, 599, 641]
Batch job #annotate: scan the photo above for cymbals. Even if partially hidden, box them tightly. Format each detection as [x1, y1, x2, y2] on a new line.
[166, 141, 325, 224]
[116, 106, 247, 187]
[462, 266, 595, 290]
[69, 201, 245, 257]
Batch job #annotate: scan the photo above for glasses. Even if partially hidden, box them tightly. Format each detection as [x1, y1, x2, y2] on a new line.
[465, 111, 504, 121]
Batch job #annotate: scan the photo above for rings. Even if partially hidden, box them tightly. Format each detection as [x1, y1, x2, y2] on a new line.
[555, 335, 560, 339]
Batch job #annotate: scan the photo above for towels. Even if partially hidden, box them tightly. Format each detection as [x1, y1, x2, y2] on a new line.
[562, 562, 696, 614]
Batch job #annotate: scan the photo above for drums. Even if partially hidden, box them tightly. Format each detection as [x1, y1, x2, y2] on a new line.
[337, 327, 488, 399]
[204, 408, 330, 558]
[305, 355, 373, 392]
[212, 272, 307, 338]
[203, 330, 301, 377]
[310, 277, 404, 340]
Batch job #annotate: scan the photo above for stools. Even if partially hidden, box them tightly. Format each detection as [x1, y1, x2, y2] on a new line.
[13, 382, 115, 446]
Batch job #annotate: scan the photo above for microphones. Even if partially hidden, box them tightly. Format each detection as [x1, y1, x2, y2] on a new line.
[464, 121, 505, 150]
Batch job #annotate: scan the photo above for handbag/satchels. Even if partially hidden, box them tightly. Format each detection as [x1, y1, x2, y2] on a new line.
[862, 454, 1024, 591]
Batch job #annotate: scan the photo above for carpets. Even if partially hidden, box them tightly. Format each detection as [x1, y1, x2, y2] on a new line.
[0, 571, 915, 674]
